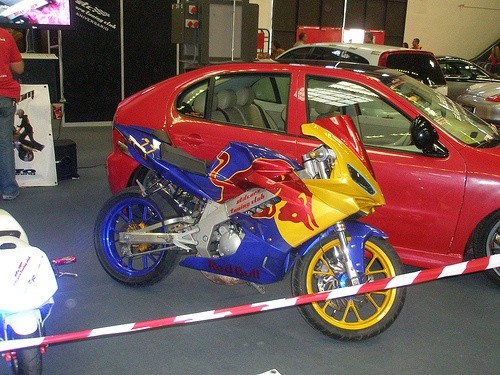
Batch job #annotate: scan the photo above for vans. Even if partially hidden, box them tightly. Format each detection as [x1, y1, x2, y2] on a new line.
[181, 41, 447, 127]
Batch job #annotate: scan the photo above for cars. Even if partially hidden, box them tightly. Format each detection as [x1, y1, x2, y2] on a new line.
[435, 54, 500, 130]
[105, 58, 500, 288]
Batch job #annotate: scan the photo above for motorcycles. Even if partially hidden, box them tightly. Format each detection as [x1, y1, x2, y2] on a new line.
[1, 208, 79, 375]
[94, 114, 409, 342]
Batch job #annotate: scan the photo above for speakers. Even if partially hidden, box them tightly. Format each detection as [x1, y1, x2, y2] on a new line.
[54, 138, 76, 180]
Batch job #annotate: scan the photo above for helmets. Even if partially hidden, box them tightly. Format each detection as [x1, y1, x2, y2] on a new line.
[16, 109, 24, 115]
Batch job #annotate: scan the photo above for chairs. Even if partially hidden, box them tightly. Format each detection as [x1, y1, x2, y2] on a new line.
[193, 87, 278, 131]
[315, 93, 343, 118]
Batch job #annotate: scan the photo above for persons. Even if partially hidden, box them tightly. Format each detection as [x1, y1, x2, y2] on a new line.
[410, 38, 422, 49]
[270, 40, 286, 59]
[363, 32, 373, 44]
[490, 43, 500, 75]
[0, 26, 24, 200]
[16, 109, 33, 143]
[294, 32, 306, 47]
[402, 43, 409, 48]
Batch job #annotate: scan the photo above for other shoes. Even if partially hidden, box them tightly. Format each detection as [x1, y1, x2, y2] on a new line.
[2, 191, 19, 200]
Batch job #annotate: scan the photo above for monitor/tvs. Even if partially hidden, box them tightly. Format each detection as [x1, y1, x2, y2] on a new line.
[0, 0, 75, 54]
